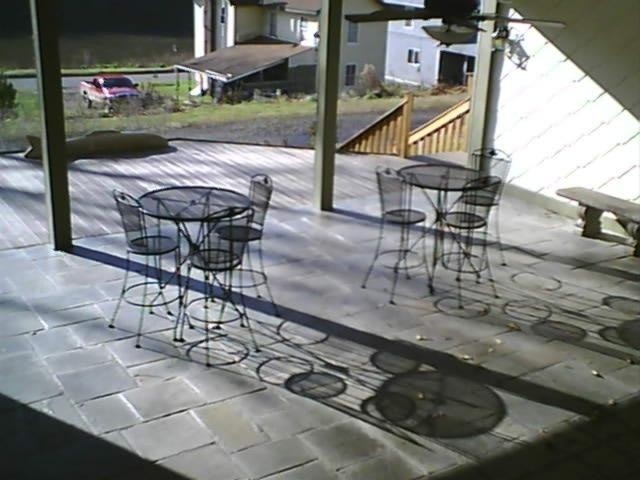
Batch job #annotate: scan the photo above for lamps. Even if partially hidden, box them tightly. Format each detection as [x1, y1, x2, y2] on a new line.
[490, 17, 511, 50]
[423, 1, 480, 46]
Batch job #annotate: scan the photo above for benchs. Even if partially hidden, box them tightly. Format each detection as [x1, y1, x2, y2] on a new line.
[556, 186, 640, 257]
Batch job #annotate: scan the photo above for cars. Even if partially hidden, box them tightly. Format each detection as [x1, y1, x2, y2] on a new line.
[77, 72, 142, 116]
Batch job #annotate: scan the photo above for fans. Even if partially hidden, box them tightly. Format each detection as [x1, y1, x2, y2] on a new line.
[344, 0, 568, 47]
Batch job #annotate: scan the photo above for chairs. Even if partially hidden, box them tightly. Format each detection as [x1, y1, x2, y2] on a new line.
[458, 147, 511, 266]
[179, 206, 260, 366]
[442, 175, 501, 309]
[205, 174, 280, 318]
[361, 165, 430, 304]
[110, 190, 181, 348]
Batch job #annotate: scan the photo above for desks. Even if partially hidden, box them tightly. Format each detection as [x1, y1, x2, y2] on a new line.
[136, 186, 251, 340]
[398, 164, 494, 287]
